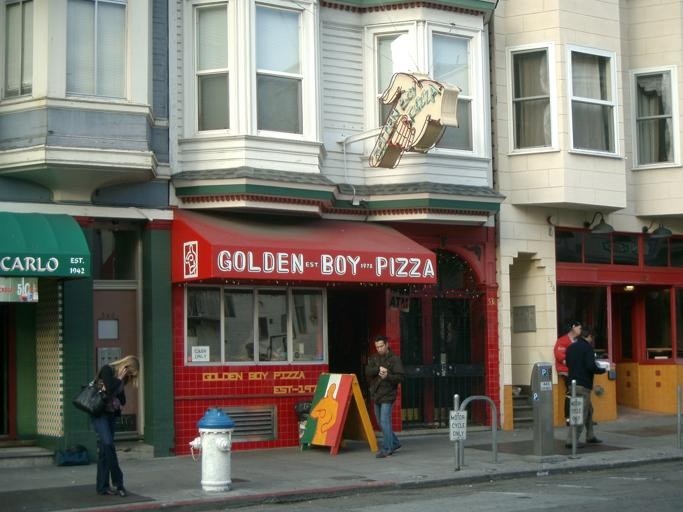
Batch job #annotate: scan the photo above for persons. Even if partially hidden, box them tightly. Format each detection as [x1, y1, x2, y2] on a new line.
[552, 319, 583, 429]
[72, 354, 140, 498]
[362, 335, 407, 460]
[563, 327, 609, 450]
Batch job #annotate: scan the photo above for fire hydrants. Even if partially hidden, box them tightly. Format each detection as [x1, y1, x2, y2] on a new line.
[188, 406, 236, 494]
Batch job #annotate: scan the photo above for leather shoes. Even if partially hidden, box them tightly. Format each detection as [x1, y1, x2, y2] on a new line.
[105, 488, 117, 495]
[117, 489, 126, 497]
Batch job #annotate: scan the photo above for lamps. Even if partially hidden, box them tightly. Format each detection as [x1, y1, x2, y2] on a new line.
[334, 182, 359, 207]
[583, 211, 674, 239]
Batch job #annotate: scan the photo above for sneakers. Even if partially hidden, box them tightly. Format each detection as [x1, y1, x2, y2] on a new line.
[565, 442, 584, 448]
[376, 444, 401, 458]
[586, 436, 602, 444]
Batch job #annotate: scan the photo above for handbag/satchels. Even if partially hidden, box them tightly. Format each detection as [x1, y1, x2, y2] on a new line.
[54, 445, 90, 466]
[72, 385, 105, 416]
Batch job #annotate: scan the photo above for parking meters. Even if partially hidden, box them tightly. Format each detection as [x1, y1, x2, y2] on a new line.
[528, 363, 555, 457]
